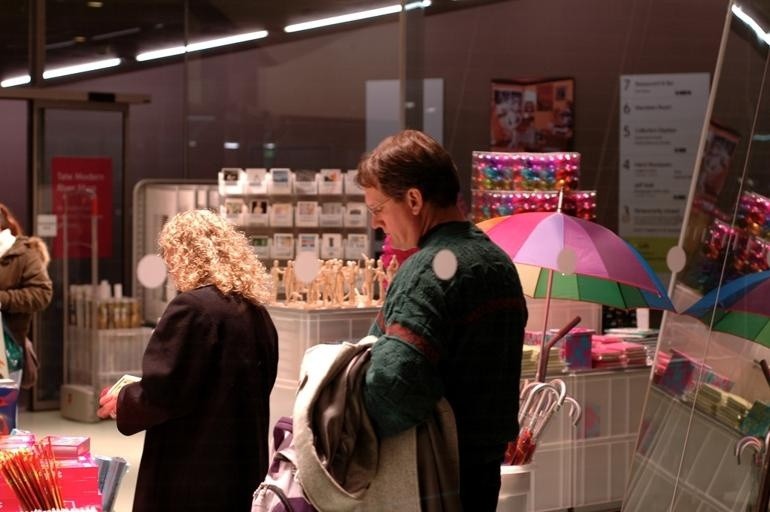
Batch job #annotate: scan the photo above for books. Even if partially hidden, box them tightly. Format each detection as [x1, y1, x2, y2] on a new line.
[220, 167, 370, 258]
[521, 326, 658, 378]
[653, 347, 770, 439]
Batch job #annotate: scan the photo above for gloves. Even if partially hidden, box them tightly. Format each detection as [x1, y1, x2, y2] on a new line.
[96, 385, 119, 422]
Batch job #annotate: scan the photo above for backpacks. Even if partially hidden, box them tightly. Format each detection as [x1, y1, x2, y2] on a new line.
[251, 417, 315, 512]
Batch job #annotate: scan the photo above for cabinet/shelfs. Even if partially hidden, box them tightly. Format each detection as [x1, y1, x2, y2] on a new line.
[217, 169, 376, 296]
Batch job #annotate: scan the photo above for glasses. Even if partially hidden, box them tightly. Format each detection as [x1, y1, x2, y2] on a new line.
[365, 198, 393, 217]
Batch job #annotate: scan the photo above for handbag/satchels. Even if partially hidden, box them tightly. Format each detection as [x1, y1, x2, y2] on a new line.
[22, 336, 41, 390]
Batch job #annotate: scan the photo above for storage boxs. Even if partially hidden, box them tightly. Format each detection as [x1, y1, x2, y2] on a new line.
[67, 326, 153, 421]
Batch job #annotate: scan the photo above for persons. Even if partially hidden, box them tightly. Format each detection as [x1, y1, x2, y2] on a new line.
[508, 100, 536, 149]
[0, 202, 55, 391]
[270, 252, 401, 309]
[344, 127, 526, 512]
[94, 208, 278, 511]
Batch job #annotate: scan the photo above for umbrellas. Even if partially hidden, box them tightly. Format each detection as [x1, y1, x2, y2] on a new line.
[474, 185, 677, 385]
[503, 379, 585, 465]
[678, 269, 769, 349]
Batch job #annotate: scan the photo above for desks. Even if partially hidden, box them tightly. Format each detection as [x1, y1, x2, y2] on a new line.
[520, 366, 652, 511]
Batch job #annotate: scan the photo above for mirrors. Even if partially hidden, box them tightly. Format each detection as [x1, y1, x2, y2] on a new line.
[623, 0, 770, 511]
